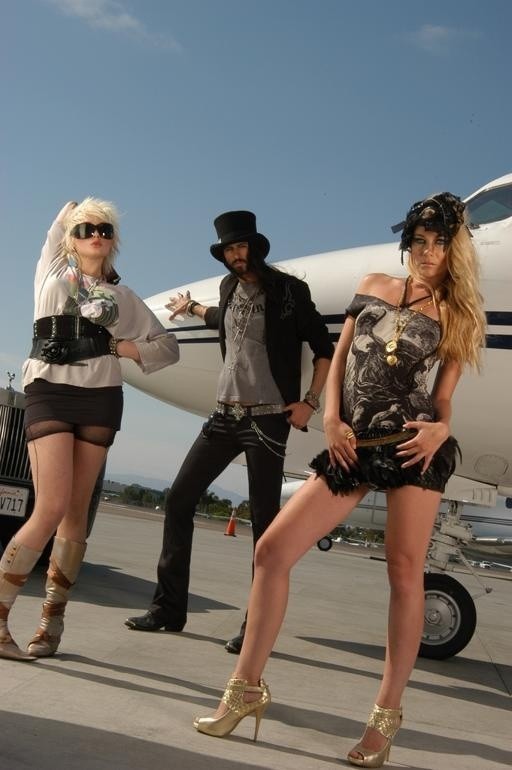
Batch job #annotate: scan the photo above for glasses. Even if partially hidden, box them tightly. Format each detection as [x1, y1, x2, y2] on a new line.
[69, 223, 116, 240]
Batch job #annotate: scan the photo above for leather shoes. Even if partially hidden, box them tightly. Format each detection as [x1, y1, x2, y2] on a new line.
[225, 620, 247, 654]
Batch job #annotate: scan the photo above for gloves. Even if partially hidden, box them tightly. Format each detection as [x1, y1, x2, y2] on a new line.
[29, 336, 112, 364]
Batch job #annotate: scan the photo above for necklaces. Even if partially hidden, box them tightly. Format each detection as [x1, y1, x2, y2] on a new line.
[385, 274, 440, 369]
[74, 269, 103, 340]
[227, 288, 259, 374]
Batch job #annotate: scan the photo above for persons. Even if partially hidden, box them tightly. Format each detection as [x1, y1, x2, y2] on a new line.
[124, 235, 336, 655]
[189, 188, 491, 769]
[1, 193, 182, 662]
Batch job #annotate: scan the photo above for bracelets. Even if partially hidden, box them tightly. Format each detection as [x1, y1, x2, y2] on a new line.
[110, 337, 123, 358]
[185, 299, 202, 319]
[302, 388, 322, 414]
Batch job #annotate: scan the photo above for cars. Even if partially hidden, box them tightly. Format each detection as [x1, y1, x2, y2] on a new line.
[0, 371, 106, 565]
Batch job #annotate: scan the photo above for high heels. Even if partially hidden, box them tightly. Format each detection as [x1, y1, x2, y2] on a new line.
[191, 676, 270, 743]
[124, 611, 187, 633]
[346, 702, 404, 768]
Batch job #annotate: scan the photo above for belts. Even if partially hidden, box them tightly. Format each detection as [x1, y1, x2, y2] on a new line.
[214, 401, 290, 420]
[357, 428, 419, 448]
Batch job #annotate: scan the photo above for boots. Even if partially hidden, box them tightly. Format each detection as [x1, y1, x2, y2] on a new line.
[0, 535, 44, 662]
[26, 535, 88, 657]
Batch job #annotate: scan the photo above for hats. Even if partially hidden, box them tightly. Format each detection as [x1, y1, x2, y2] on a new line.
[209, 210, 271, 264]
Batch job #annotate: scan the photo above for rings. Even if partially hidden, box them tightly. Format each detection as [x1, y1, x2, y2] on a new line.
[344, 431, 355, 440]
